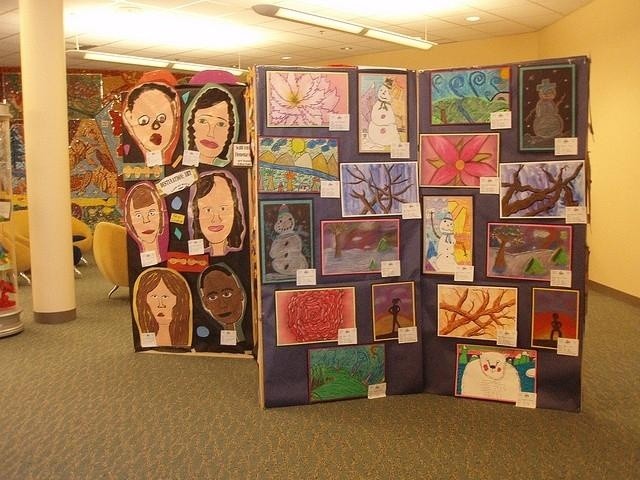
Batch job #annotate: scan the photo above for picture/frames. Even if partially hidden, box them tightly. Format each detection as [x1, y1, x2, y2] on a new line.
[417, 133, 500, 191]
[421, 194, 476, 277]
[307, 340, 387, 405]
[265, 69, 350, 128]
[531, 288, 580, 351]
[517, 66, 577, 153]
[315, 215, 402, 283]
[434, 281, 519, 345]
[497, 159, 586, 220]
[484, 221, 574, 283]
[428, 66, 512, 127]
[339, 160, 420, 218]
[371, 280, 417, 343]
[357, 72, 408, 153]
[274, 286, 356, 349]
[260, 135, 339, 195]
[260, 197, 317, 286]
[454, 343, 539, 406]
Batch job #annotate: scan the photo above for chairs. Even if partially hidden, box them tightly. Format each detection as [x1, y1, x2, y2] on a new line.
[0, 210, 130, 301]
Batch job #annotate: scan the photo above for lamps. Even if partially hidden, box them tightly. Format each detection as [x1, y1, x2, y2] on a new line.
[64, 1, 276, 55]
[252, 2, 439, 51]
[64, 40, 249, 77]
